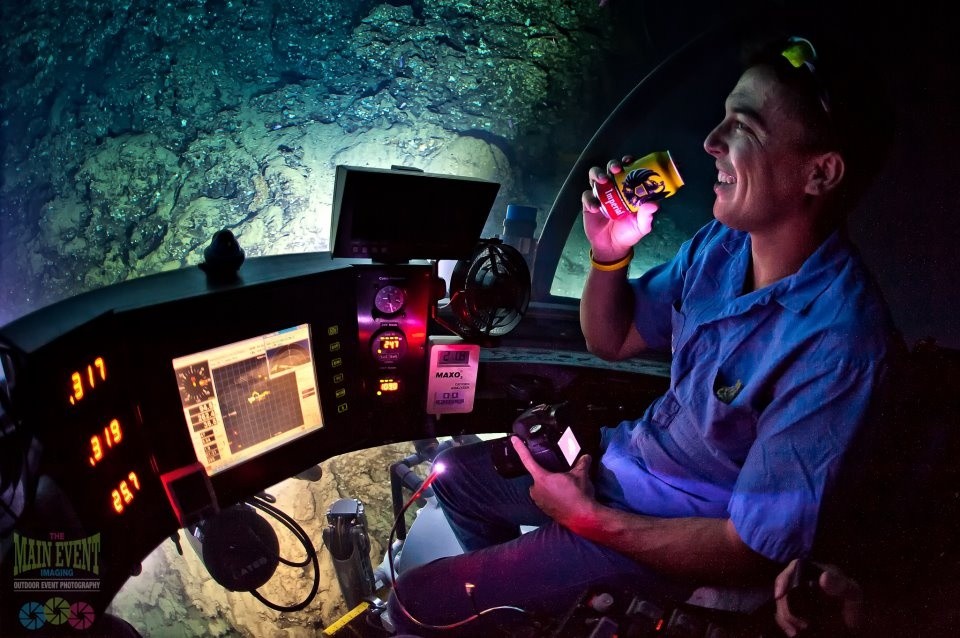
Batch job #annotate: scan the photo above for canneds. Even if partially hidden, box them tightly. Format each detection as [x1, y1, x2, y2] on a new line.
[593, 150, 684, 220]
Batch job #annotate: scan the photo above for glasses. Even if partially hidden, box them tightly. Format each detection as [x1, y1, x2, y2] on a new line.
[776, 36, 841, 150]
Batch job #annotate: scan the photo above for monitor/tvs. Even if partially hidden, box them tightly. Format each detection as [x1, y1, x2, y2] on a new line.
[173, 323, 323, 477]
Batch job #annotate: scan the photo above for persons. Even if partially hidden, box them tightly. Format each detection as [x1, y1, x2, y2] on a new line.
[775, 558, 960, 638]
[386, 37, 910, 638]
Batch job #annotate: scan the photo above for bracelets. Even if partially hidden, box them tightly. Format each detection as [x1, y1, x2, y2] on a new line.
[590, 246, 634, 272]
